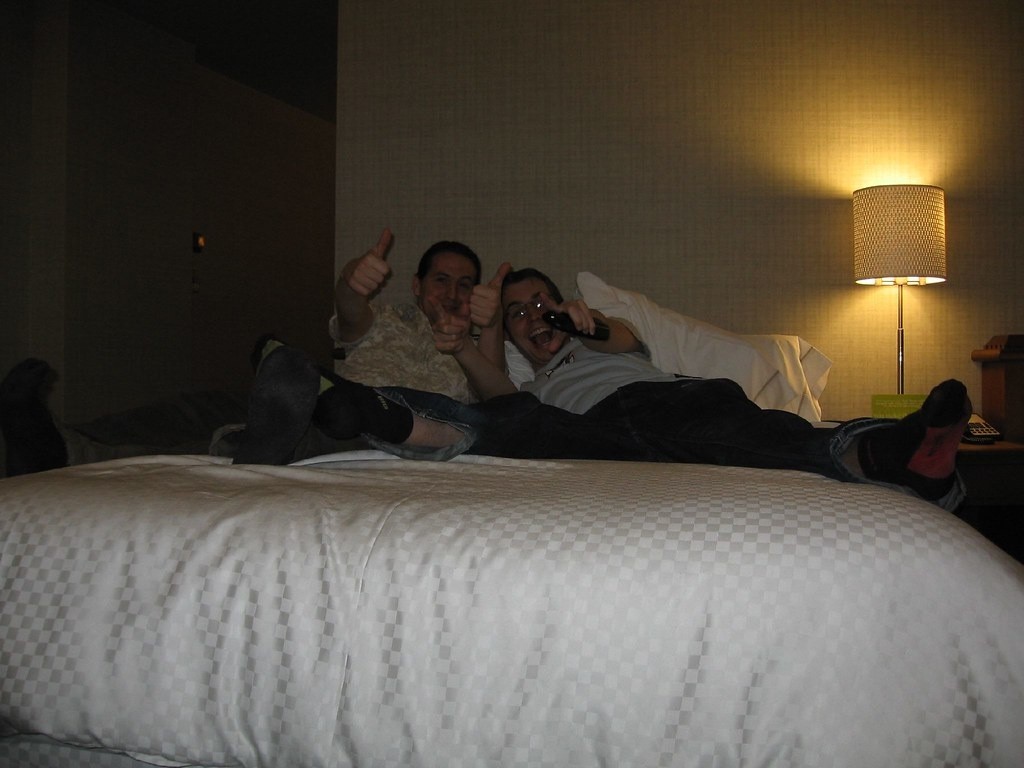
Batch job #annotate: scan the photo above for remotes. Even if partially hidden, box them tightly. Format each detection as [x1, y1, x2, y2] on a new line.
[541, 310, 610, 341]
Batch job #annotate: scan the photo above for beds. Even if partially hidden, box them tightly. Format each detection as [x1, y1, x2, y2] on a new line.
[1, 453, 1024, 768]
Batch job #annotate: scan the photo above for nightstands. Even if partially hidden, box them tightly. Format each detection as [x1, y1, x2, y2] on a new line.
[955, 439, 1024, 561]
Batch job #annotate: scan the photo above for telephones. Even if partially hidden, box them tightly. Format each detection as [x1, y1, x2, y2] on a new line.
[960, 412, 1000, 444]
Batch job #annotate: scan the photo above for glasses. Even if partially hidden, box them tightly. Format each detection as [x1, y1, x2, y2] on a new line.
[502, 295, 560, 321]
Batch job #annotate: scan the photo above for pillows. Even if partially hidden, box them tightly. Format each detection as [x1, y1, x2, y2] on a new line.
[503, 270, 831, 424]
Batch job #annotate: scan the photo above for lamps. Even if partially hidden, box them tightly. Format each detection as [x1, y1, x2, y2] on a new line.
[853, 184, 947, 393]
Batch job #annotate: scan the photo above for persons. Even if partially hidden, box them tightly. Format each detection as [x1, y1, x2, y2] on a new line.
[0, 227, 512, 478]
[251, 268, 973, 501]
